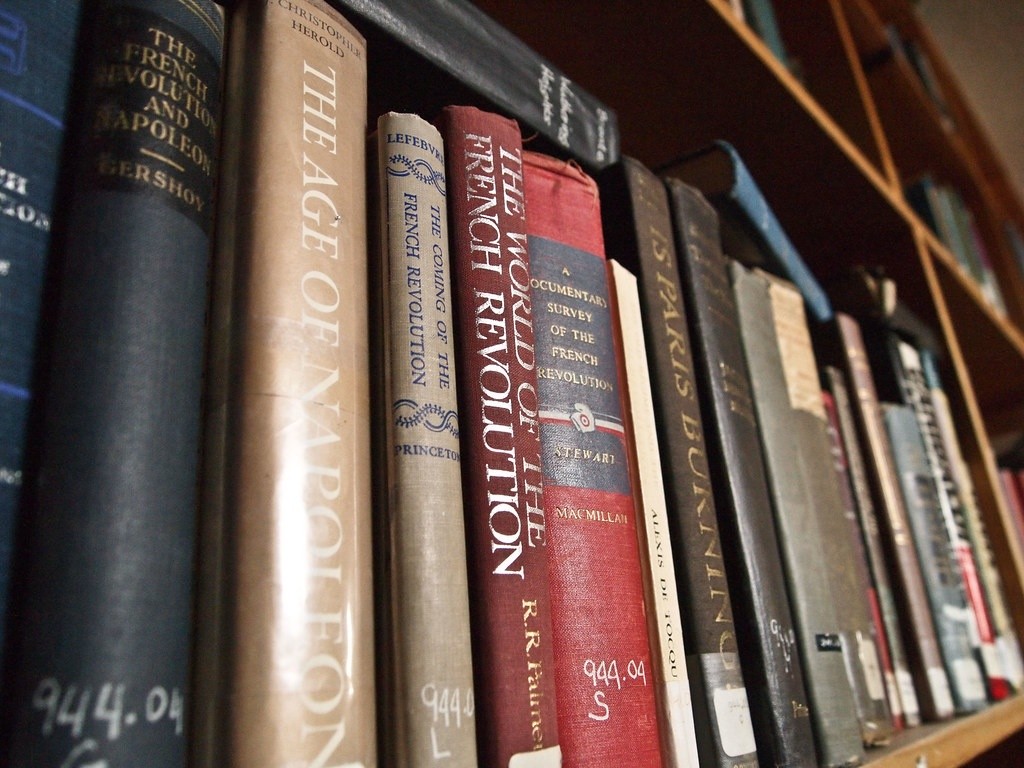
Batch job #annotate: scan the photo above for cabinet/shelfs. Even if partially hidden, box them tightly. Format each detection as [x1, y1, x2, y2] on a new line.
[472, 0, 1024, 768]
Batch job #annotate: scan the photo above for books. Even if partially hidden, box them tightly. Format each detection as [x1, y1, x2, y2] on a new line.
[0, 0, 1024, 768]
